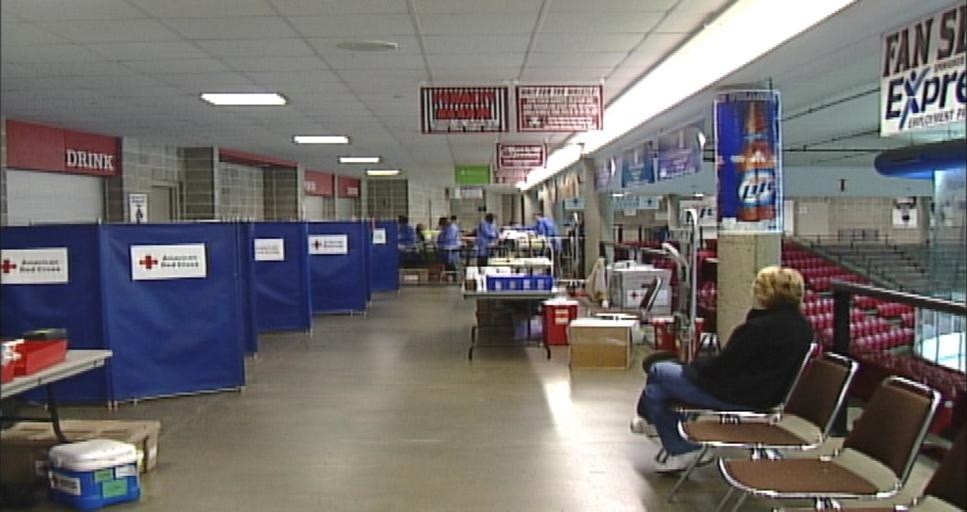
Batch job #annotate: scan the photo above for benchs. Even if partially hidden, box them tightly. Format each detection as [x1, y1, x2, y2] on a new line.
[814, 240, 967, 292]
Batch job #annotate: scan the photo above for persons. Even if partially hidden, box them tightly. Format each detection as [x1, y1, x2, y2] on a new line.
[627, 263, 816, 477]
[399, 211, 464, 283]
[474, 212, 496, 271]
[531, 211, 561, 284]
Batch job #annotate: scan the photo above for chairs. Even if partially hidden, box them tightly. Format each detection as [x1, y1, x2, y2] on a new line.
[717, 376, 943, 510]
[595, 278, 664, 321]
[782, 242, 916, 352]
[656, 344, 859, 502]
[880, 424, 966, 512]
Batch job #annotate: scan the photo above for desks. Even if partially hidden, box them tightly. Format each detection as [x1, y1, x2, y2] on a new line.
[1, 349, 113, 445]
[463, 290, 555, 360]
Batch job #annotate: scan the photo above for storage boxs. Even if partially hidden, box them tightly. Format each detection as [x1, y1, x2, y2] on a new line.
[568, 319, 635, 371]
[0, 419, 161, 511]
[542, 300, 579, 346]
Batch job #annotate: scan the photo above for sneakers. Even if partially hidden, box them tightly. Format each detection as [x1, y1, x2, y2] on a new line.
[654, 443, 716, 474]
[631, 415, 658, 437]
